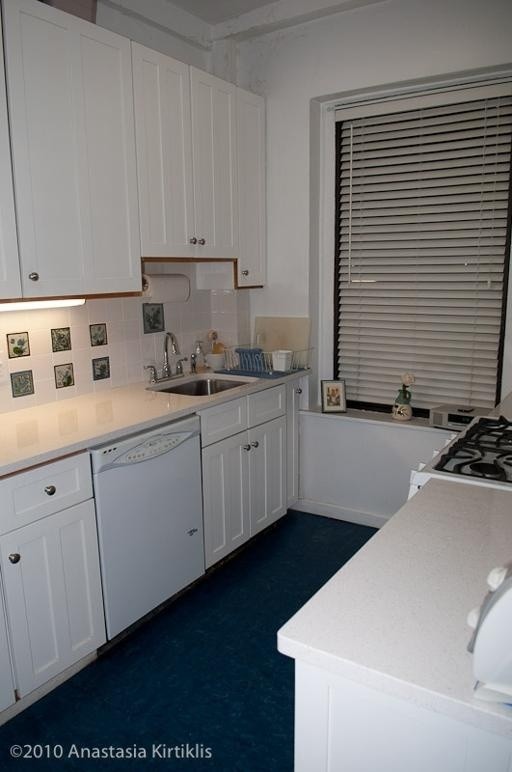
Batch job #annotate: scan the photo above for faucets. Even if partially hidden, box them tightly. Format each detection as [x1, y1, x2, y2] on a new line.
[162, 332, 177, 378]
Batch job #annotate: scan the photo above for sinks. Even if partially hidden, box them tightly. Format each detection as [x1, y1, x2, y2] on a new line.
[143, 373, 258, 397]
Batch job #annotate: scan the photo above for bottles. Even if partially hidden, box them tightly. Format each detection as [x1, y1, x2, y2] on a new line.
[194, 343, 204, 371]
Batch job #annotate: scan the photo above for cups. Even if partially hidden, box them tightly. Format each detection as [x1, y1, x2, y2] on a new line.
[207, 354, 225, 371]
[271, 350, 293, 372]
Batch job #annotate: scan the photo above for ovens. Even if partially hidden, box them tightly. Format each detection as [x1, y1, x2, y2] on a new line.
[91, 413, 209, 644]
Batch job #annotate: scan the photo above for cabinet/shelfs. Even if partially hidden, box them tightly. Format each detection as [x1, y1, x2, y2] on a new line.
[0, 1, 146, 308]
[196, 373, 291, 574]
[122, 36, 238, 261]
[0, 442, 109, 726]
[195, 71, 272, 293]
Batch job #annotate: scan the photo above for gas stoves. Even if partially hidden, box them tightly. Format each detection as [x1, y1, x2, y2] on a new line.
[408, 414, 512, 497]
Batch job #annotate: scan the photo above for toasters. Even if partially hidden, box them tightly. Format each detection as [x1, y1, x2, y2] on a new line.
[467, 561, 512, 694]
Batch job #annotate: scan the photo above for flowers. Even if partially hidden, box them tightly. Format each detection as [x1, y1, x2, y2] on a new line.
[399, 370, 417, 392]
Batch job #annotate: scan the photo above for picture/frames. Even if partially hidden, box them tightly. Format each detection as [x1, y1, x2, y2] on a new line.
[318, 379, 347, 415]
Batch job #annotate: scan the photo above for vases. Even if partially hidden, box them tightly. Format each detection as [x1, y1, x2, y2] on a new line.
[388, 386, 414, 422]
